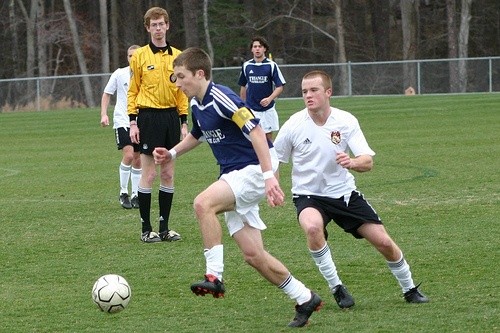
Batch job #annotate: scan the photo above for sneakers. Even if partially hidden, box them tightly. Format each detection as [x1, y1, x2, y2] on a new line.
[158, 230, 182, 241]
[191, 274, 225, 299]
[287, 291, 324, 327]
[331, 284, 355, 309]
[141, 231, 162, 242]
[119, 193, 132, 209]
[404, 283, 429, 303]
[131, 194, 140, 208]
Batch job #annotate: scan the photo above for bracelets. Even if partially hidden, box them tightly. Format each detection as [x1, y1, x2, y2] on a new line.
[168, 148, 176, 160]
[262, 170, 274, 180]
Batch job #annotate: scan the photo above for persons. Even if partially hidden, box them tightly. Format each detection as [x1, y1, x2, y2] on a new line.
[126, 6, 191, 244]
[151, 46, 322, 329]
[101, 45, 148, 208]
[270, 70, 429, 307]
[238, 39, 285, 144]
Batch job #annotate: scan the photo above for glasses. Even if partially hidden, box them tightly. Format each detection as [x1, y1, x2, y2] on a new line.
[148, 22, 167, 27]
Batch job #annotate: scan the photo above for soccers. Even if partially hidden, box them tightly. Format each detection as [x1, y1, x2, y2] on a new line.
[90, 273, 132, 314]
